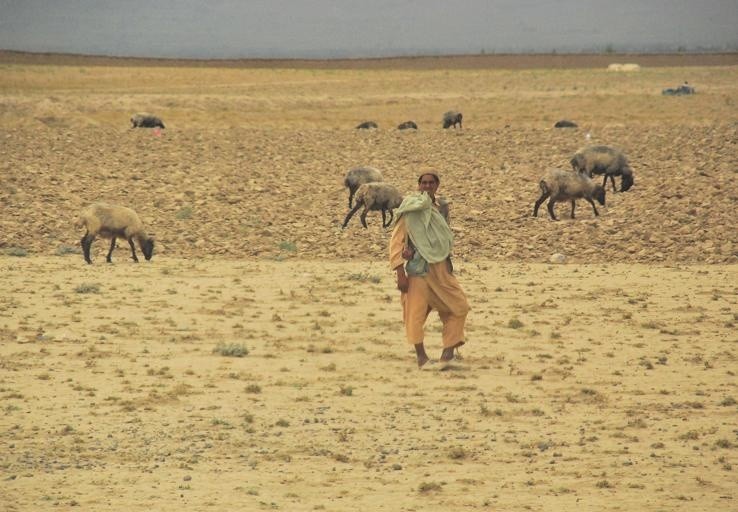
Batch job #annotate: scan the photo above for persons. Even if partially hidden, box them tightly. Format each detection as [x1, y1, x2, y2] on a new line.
[389, 169, 472, 370]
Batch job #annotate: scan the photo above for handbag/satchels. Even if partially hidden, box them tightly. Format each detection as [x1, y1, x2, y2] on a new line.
[406, 251, 428, 276]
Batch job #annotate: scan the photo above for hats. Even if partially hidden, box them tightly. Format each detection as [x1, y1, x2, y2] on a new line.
[418, 166, 439, 182]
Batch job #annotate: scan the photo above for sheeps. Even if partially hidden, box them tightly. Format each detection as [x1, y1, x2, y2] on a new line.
[75, 201, 153, 264]
[533, 169, 606, 221]
[345, 166, 384, 210]
[399, 121, 418, 130]
[554, 119, 578, 128]
[442, 112, 463, 130]
[358, 120, 378, 129]
[342, 182, 403, 228]
[129, 113, 166, 130]
[570, 144, 633, 193]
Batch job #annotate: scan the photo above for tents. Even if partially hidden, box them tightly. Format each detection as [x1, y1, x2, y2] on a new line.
[623, 64, 640, 72]
[607, 64, 624, 71]
